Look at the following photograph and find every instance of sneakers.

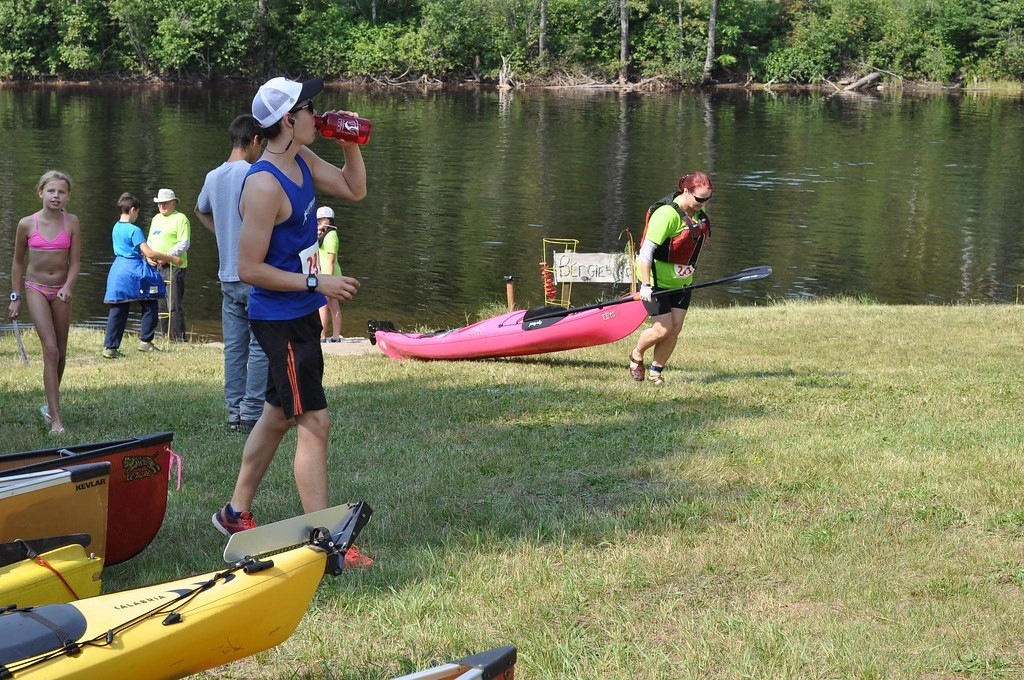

[212,502,255,540]
[343,543,374,568]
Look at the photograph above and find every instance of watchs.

[10,293,22,301]
[305,273,318,292]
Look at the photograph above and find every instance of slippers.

[48,428,65,438]
[45,413,52,426]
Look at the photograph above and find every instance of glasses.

[289,100,313,114]
[688,190,712,203]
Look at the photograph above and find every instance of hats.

[251,76,323,129]
[153,188,181,206]
[316,205,335,220]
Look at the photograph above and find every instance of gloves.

[639,287,658,303]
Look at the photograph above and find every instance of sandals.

[628,351,645,381]
[647,373,664,387]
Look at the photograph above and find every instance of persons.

[101,191,184,359]
[314,206,343,342]
[145,188,191,345]
[193,115,265,434]
[8,171,80,438]
[629,171,712,387]
[210,77,376,574]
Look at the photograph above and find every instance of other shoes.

[102,348,126,359]
[320,339,327,344]
[239,419,259,434]
[229,422,239,432]
[137,341,161,352]
[327,337,342,343]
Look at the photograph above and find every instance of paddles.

[522,266,773,332]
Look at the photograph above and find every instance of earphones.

[288,117,295,125]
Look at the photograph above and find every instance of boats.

[366,291,648,360]
[1,433,175,611]
[0,503,518,680]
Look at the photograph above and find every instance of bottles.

[315,112,373,144]
